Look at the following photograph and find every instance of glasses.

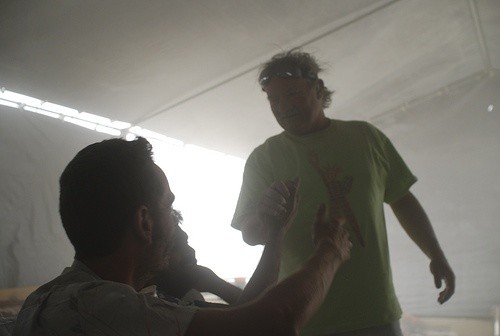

[261,70,316,91]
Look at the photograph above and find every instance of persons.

[11,136,353,336]
[231,47,456,336]
